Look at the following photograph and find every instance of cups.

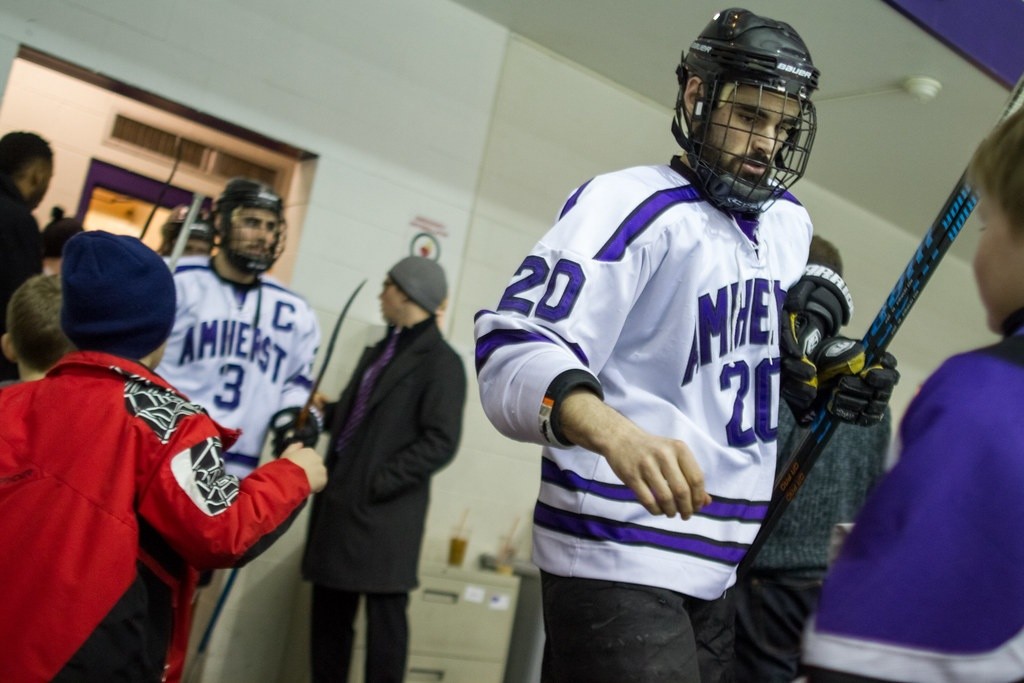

[448,528,470,566]
[497,535,521,574]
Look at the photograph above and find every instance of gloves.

[777,261,853,427]
[808,335,902,429]
[270,405,322,456]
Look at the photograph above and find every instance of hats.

[389,255,446,314]
[61,229,175,359]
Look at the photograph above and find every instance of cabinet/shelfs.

[280,560,520,683]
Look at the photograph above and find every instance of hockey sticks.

[173,278,371,683]
[694,74,1024,636]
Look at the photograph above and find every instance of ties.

[337,332,399,453]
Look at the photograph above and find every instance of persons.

[42,208,85,275]
[149,178,322,480]
[0,231,327,683]
[1,273,76,382]
[0,132,52,378]
[472,7,899,683]
[803,102,1024,683]
[727,236,891,683]
[300,256,466,683]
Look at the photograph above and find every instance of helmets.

[668,6,821,212]
[215,177,285,272]
[157,206,215,257]
